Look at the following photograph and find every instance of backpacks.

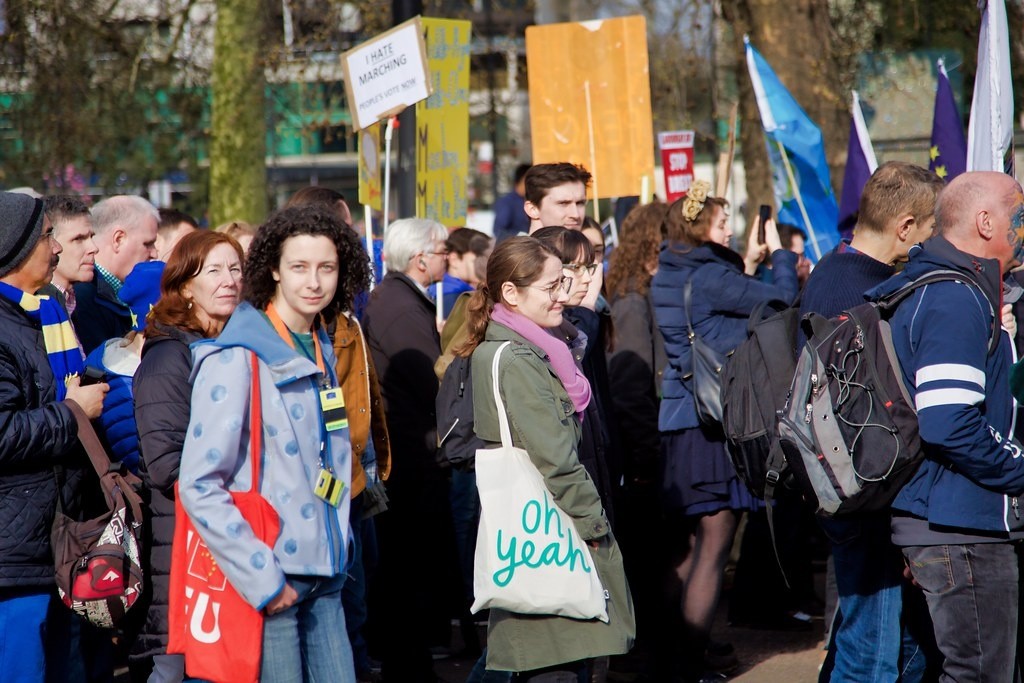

[719,248,834,500]
[763,270,996,544]
[435,354,481,465]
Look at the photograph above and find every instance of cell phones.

[758,204,771,244]
[79,365,108,387]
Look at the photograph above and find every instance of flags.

[928,57,966,180]
[745,44,879,263]
[965,0,1015,178]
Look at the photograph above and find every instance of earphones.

[420,259,427,267]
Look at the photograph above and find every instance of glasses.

[516,277,573,301]
[426,249,449,260]
[561,263,597,277]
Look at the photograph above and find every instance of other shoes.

[788,605,825,620]
[702,631,734,655]
[647,653,739,679]
[752,612,813,630]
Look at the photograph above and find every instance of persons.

[0,163,1024,683]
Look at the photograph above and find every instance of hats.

[0,192,43,277]
[6,187,44,198]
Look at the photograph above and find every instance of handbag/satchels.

[166,349,282,683]
[50,398,143,627]
[469,341,610,622]
[684,271,728,443]
